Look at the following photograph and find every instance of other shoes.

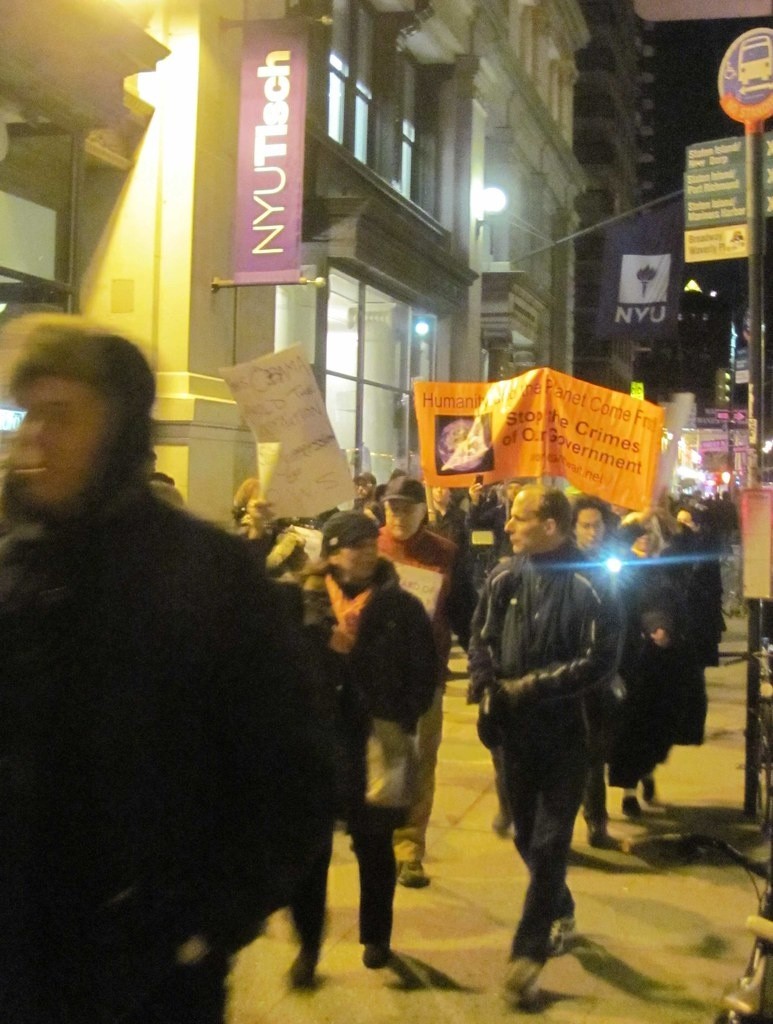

[641,772,655,802]
[362,943,389,969]
[500,955,544,1007]
[397,860,425,888]
[587,820,607,847]
[548,916,583,956]
[620,789,643,818]
[290,949,318,987]
[493,812,511,832]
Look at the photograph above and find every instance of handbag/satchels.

[366,735,425,808]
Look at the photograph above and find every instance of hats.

[320,510,382,558]
[380,476,427,505]
[352,471,377,485]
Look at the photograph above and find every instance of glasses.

[355,482,367,489]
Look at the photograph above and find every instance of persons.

[1,330,333,1023]
[233,469,742,1004]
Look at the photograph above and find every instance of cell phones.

[475,474,484,486]
